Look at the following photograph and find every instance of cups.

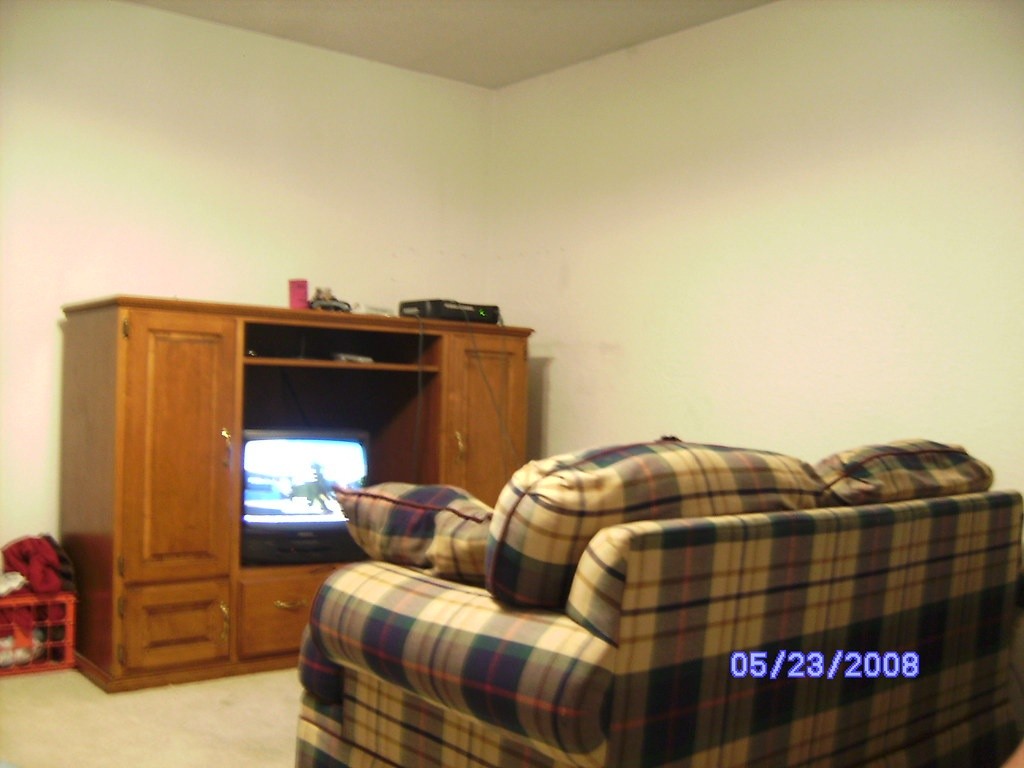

[288,278,310,311]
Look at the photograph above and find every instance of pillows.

[482,434,825,618]
[334,481,493,589]
[816,439,994,506]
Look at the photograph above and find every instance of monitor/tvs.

[240,428,373,564]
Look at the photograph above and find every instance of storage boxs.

[0,592,76,676]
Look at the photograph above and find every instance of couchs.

[295,490,1024,768]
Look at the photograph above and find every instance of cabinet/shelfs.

[59,293,535,696]
